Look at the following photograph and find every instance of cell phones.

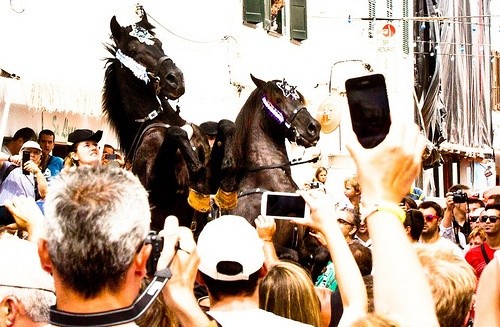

[260,191,310,221]
[345,73,391,150]
[22,151,30,176]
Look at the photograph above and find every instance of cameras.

[311,182,319,189]
[452,193,467,203]
[145,230,164,275]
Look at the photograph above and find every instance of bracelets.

[360,201,406,223]
[8,156,11,162]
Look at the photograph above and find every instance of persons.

[132,190,368,327]
[345,114,499,327]
[1,233,56,327]
[308,164,327,191]
[36,165,216,327]
[0,127,124,240]
[256,0,285,31]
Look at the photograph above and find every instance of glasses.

[337,218,354,226]
[482,215,500,223]
[136,231,157,254]
[423,214,436,222]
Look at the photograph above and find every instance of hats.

[68,128,103,144]
[20,141,42,151]
[196,215,265,281]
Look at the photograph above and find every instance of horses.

[206,72,323,271]
[97,6,240,284]
